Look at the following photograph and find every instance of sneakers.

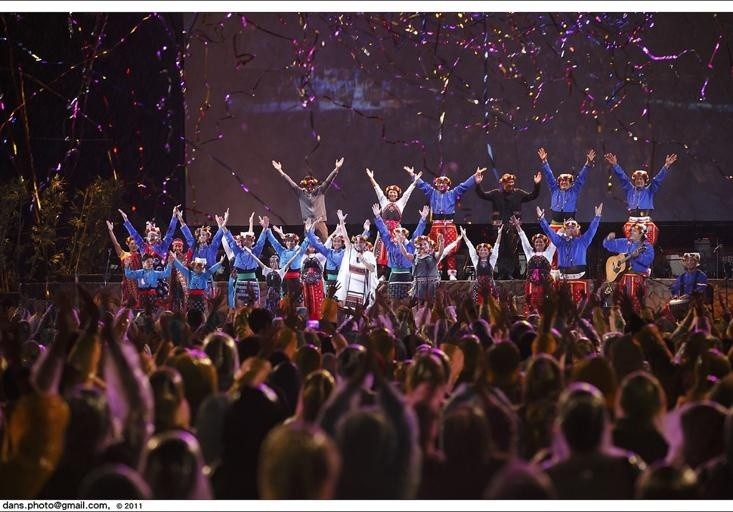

[377,274,387,283]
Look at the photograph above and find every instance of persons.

[537,147,597,269]
[364,168,422,264]
[271,157,344,242]
[403,165,487,280]
[604,153,678,243]
[473,171,541,279]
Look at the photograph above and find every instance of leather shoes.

[496,272,515,280]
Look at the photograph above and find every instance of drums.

[669,298,689,317]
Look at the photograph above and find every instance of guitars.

[605,246,646,283]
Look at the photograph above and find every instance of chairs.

[666,255,687,279]
[75,245,112,288]
[694,238,725,279]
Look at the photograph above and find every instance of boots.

[446,268,459,282]
